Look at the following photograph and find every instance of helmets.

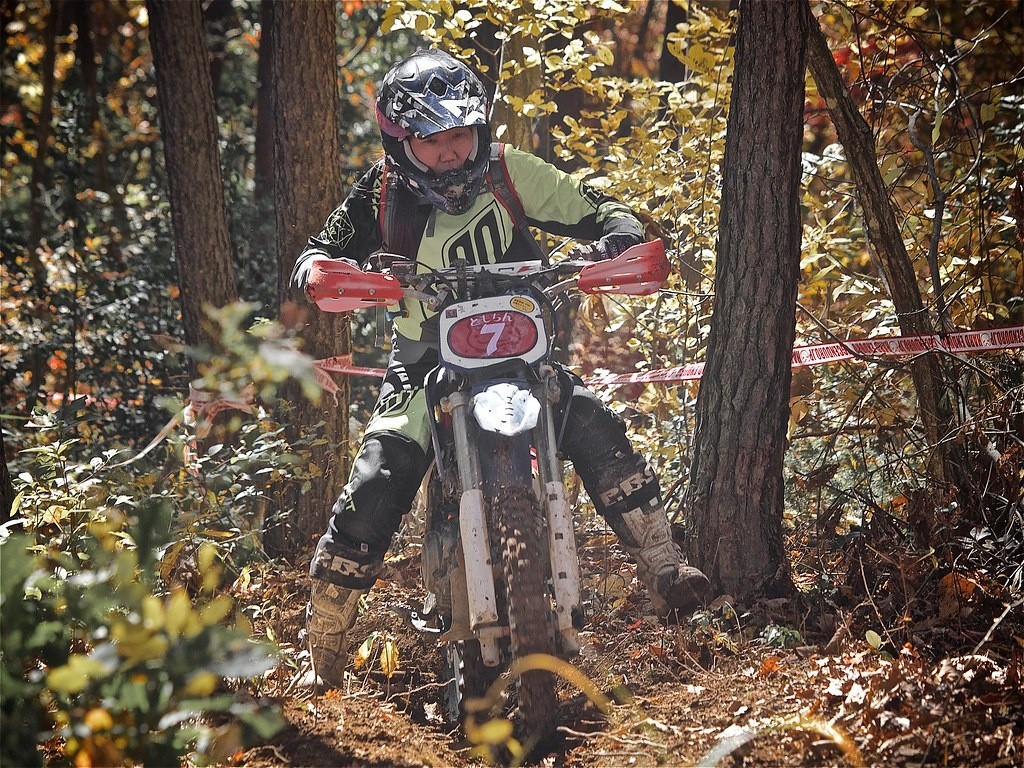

[374,48,492,216]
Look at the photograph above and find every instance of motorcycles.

[305,235,670,721]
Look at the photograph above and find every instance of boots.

[606,490,710,628]
[297,576,368,694]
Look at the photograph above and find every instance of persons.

[289,49,710,695]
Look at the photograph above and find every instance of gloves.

[336,257,359,269]
[597,233,640,261]
